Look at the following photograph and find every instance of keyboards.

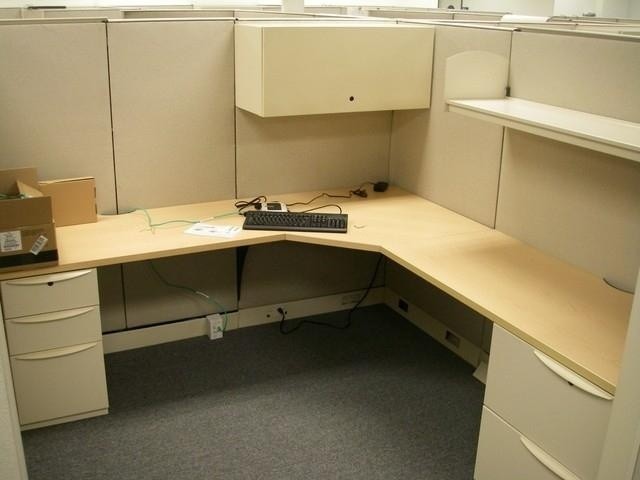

[243,211,348,232]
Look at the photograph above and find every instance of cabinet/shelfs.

[234,20,436,120]
[2,269,113,433]
[472,323,613,480]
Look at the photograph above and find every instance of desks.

[0,183,633,396]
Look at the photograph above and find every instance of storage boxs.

[1,167,59,273]
